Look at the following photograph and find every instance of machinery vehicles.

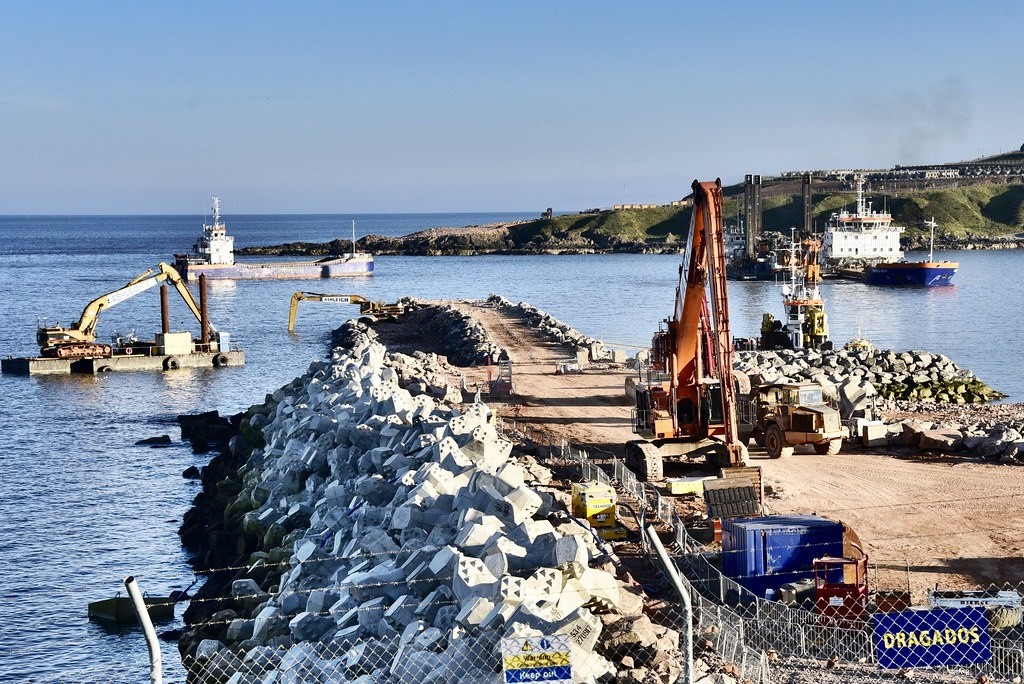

[36,261,220,358]
[288,291,390,331]
[624,175,765,513]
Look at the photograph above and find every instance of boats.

[170,197,375,281]
[817,175,960,289]
[721,172,823,286]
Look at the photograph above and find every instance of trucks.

[735,378,850,462]
